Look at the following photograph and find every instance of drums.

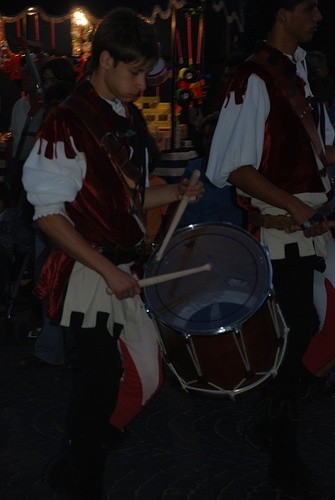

[143,219,291,398]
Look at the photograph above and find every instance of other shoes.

[45,453,106,499]
[269,455,322,500]
[245,413,267,450]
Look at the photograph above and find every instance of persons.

[21,8,205,500]
[0,32,335,401]
[204,0,335,500]
